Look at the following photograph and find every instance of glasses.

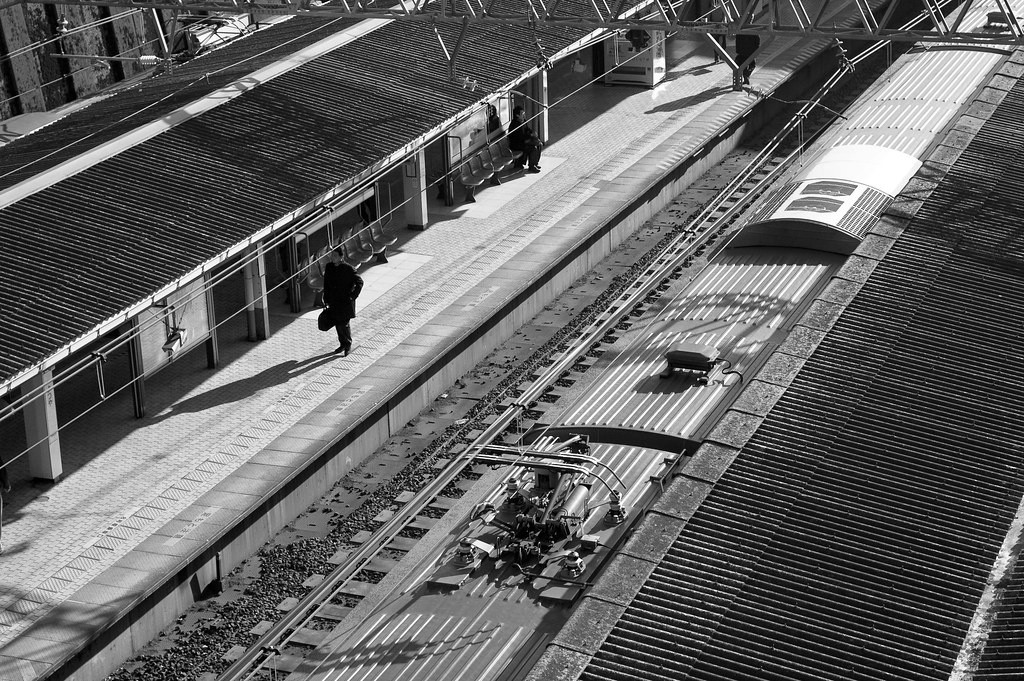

[520,112,526,115]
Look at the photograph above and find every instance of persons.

[505,104,544,175]
[317,245,362,359]
[487,105,500,136]
[713,32,729,64]
[736,30,763,87]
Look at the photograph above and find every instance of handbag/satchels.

[525,128,540,145]
[318,304,335,331]
[734,53,742,66]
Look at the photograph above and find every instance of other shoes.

[529,166,540,172]
[715,60,719,63]
[536,166,541,169]
[744,75,749,84]
[345,349,349,356]
[335,346,345,353]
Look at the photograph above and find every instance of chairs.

[370,222,398,264]
[469,157,495,179]
[498,138,523,159]
[317,253,329,276]
[458,165,484,203]
[479,150,505,185]
[344,237,374,263]
[488,144,514,165]
[306,256,326,308]
[358,229,386,254]
[330,245,361,268]
